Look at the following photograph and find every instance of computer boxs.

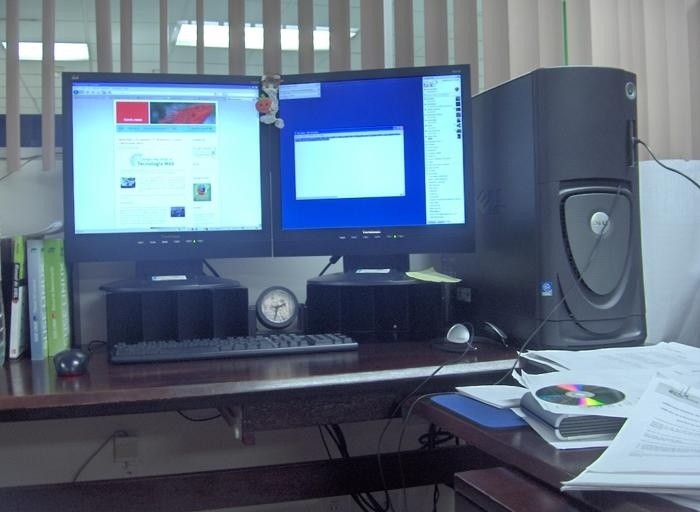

[445,66,647,349]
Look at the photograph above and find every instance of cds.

[535,383,625,409]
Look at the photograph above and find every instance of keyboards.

[108,331,361,365]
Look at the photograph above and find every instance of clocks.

[253,283,300,330]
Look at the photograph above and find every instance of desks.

[2,339,535,429]
[415,388,698,510]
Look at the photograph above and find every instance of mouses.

[53,348,90,377]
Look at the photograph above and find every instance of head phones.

[433,319,511,353]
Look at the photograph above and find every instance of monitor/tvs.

[272,64,477,286]
[60,71,273,291]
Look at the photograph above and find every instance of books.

[8,232,28,360]
[511,337,700,493]
[41,229,72,358]
[0,234,14,359]
[22,222,63,362]
[0,356,61,397]
